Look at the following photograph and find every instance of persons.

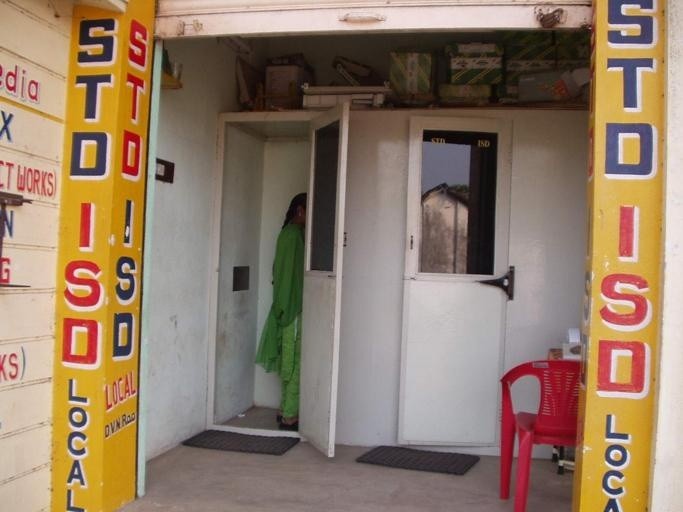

[252,192,307,431]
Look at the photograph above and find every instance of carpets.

[356,445,481,476]
[182,429,300,455]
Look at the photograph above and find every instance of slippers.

[277,411,299,431]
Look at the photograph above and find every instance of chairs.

[499,360,584,512]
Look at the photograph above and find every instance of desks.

[546,345,583,475]
